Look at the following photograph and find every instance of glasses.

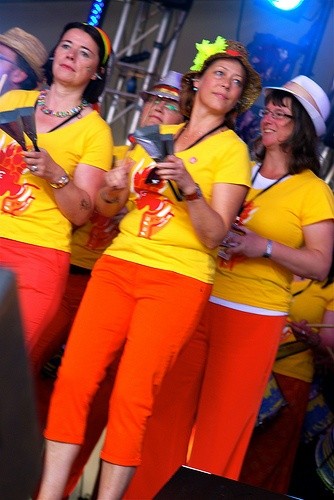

[259,108,295,120]
[148,96,179,111]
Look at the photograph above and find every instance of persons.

[119,70,333,500]
[0,23,49,93]
[236,255,333,494]
[32,29,263,500]
[0,19,116,460]
[23,68,189,499]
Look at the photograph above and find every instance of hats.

[178,35,261,119]
[0,27,49,84]
[262,74,331,138]
[139,70,188,103]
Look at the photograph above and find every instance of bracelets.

[262,237,280,264]
[48,163,71,193]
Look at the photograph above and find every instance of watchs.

[180,176,206,207]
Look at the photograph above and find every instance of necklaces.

[261,169,280,180]
[181,120,209,143]
[36,87,89,120]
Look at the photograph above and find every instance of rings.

[30,166,37,173]
[301,331,306,335]
